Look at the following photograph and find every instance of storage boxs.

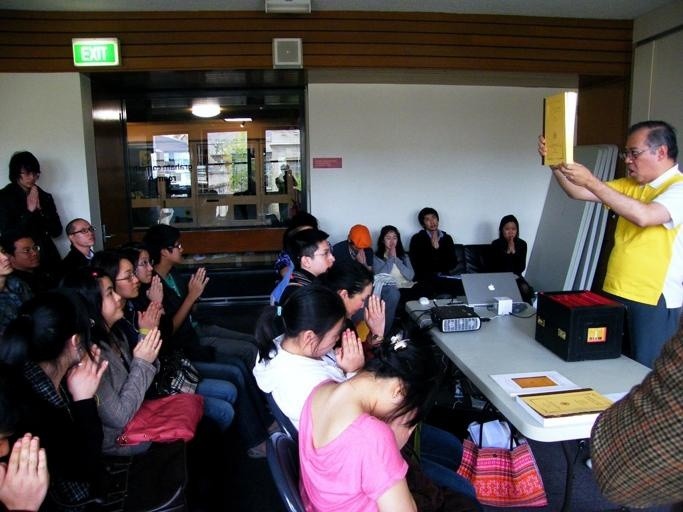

[532,287,637,362]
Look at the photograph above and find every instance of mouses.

[418,297,429,305]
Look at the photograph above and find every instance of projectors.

[430,305,481,333]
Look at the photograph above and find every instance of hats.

[348,225,373,249]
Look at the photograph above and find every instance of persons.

[408,208,464,296]
[537,120,682,368]
[487,214,533,306]
[0,150,63,269]
[1,219,474,510]
[274,163,298,219]
[589,306,681,512]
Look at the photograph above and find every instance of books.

[515,387,613,427]
[488,369,579,398]
[539,90,579,167]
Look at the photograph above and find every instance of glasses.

[16,245,41,253]
[73,226,97,234]
[163,242,185,250]
[114,270,139,282]
[135,259,155,268]
[619,146,651,159]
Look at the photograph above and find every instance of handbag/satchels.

[455,401,549,509]
[116,393,203,444]
[153,357,201,398]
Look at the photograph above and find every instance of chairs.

[264,390,299,440]
[264,427,307,512]
[464,243,493,271]
[450,242,465,276]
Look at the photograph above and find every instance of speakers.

[493,296,513,316]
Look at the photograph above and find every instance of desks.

[404,295,656,512]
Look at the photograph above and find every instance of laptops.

[457,272,524,307]
